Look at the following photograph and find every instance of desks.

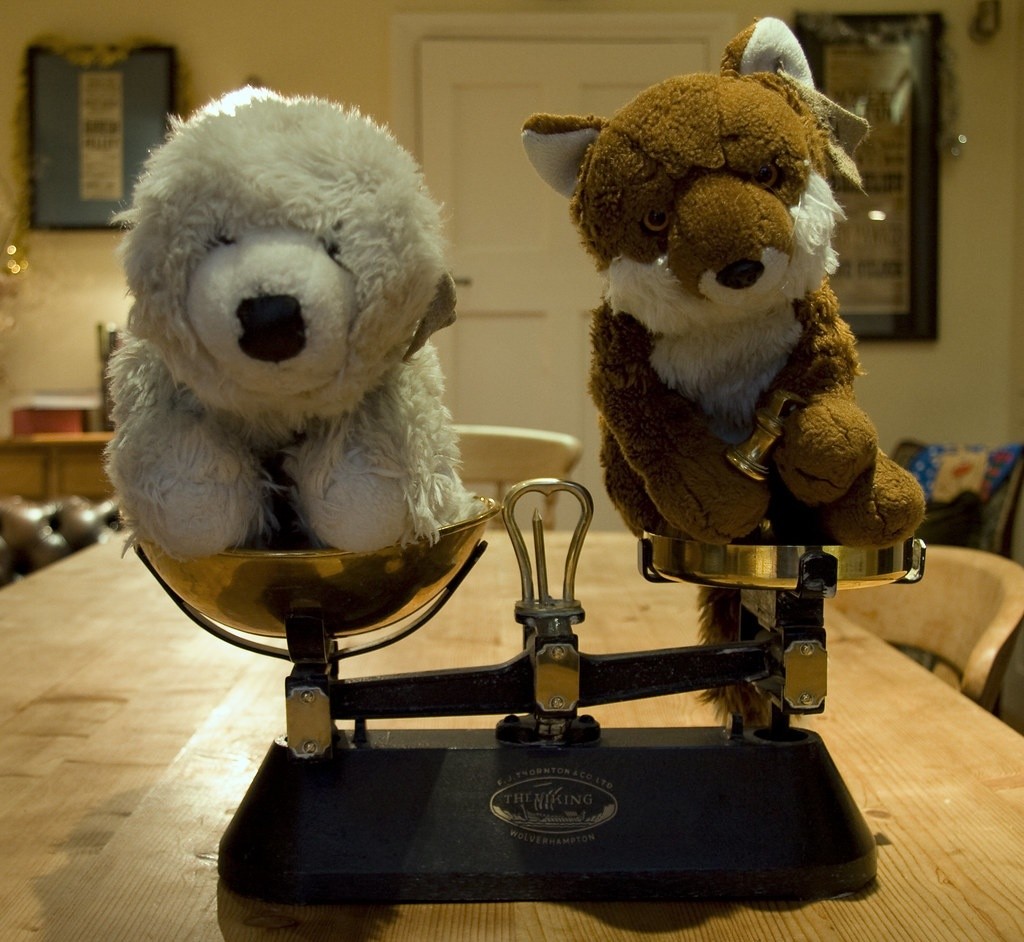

[0,531,1023,942]
[0,432,115,501]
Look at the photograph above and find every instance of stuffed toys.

[106,84,480,556]
[519,14,928,726]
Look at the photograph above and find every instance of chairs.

[449,425,582,535]
[830,545,1022,711]
[892,440,1024,556]
